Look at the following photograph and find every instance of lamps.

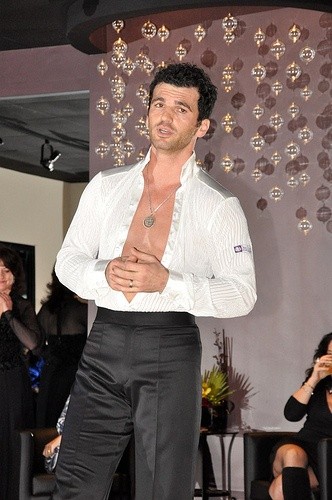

[40,138,63,171]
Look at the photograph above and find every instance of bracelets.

[302,383,315,395]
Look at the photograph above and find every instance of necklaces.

[143,161,182,229]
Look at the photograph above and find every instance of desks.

[199,429,243,500]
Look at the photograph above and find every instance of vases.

[201,401,213,429]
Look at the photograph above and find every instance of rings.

[129,277,134,287]
[48,450,50,453]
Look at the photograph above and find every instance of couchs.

[243,431,332,500]
[19,428,135,500]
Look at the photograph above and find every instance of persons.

[43,394,137,500]
[0,245,43,500]
[35,261,83,476]
[49,62,258,500]
[268,331,332,500]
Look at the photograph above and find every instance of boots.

[282,467,311,500]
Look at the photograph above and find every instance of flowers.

[200,368,235,406]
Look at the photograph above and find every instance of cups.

[325,357,332,375]
